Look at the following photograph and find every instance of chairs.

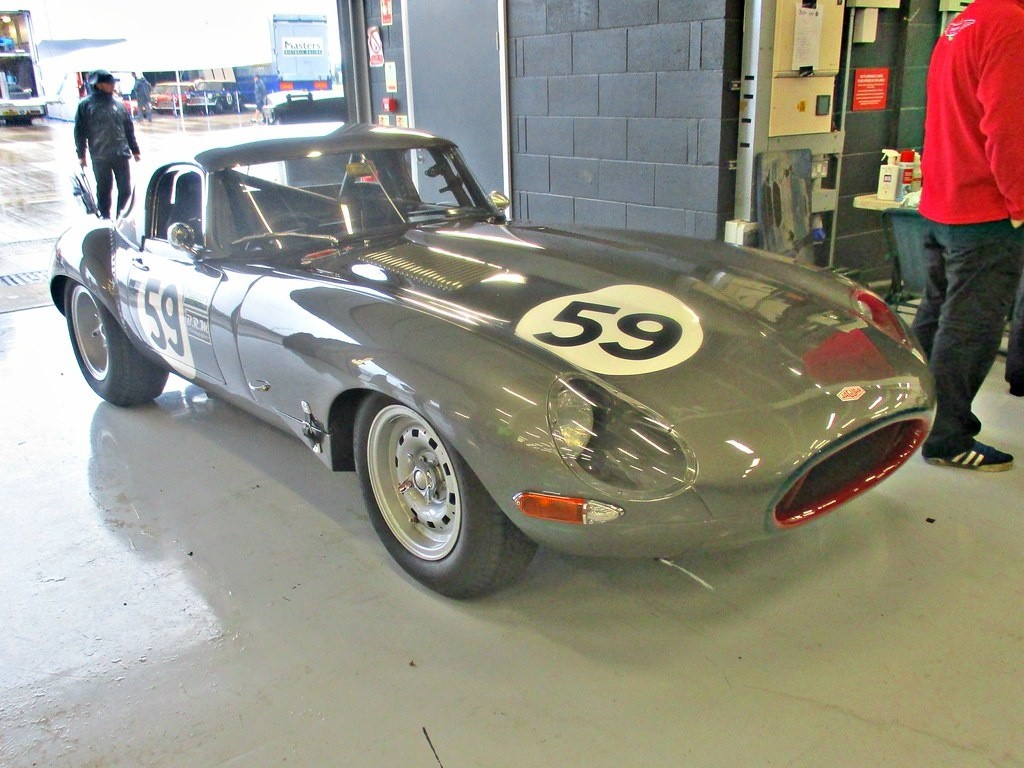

[167,170,241,257]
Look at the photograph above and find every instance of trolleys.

[880,209,937,313]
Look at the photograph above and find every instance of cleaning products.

[876,148,922,202]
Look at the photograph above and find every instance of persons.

[133,76,152,122]
[911,0,1024,471]
[73,70,140,218]
[251,74,269,122]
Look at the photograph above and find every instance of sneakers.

[923,437,1013,472]
[967,411,980,438]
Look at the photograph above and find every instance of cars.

[186,80,242,114]
[149,83,194,116]
[47,123,938,599]
[261,91,307,126]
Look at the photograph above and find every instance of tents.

[69,34,273,118]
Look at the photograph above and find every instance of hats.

[95,71,120,84]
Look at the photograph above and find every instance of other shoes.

[1010,384,1024,397]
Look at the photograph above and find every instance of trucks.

[0,10,48,126]
[43,68,154,120]
